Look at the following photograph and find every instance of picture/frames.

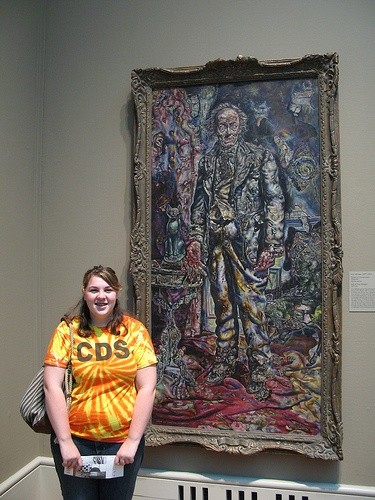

[130,52,345,462]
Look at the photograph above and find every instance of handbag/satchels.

[19,363,72,433]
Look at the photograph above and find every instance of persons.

[41,264,159,499]
